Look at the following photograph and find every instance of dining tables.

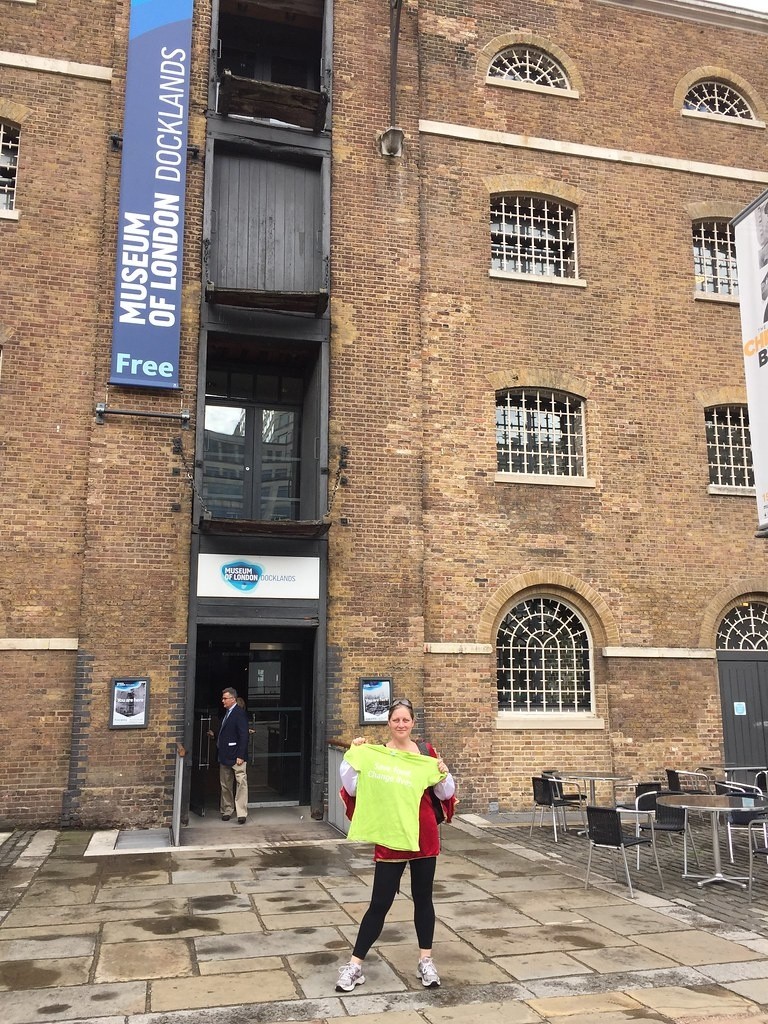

[700,765,767,826]
[654,795,768,891]
[552,771,634,837]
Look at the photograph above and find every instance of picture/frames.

[357,677,395,726]
[108,676,149,730]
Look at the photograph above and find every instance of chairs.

[529,776,589,845]
[585,805,665,901]
[616,781,664,849]
[713,779,768,850]
[665,768,721,832]
[632,790,702,879]
[539,769,588,836]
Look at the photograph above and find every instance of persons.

[207,687,250,824]
[335,699,455,993]
[126,688,134,716]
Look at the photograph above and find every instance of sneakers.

[416,956,442,987]
[335,965,366,992]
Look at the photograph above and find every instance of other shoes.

[238,817,246,823]
[222,815,230,820]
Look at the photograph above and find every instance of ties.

[222,710,230,727]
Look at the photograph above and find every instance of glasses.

[221,697,231,700]
[392,699,412,708]
